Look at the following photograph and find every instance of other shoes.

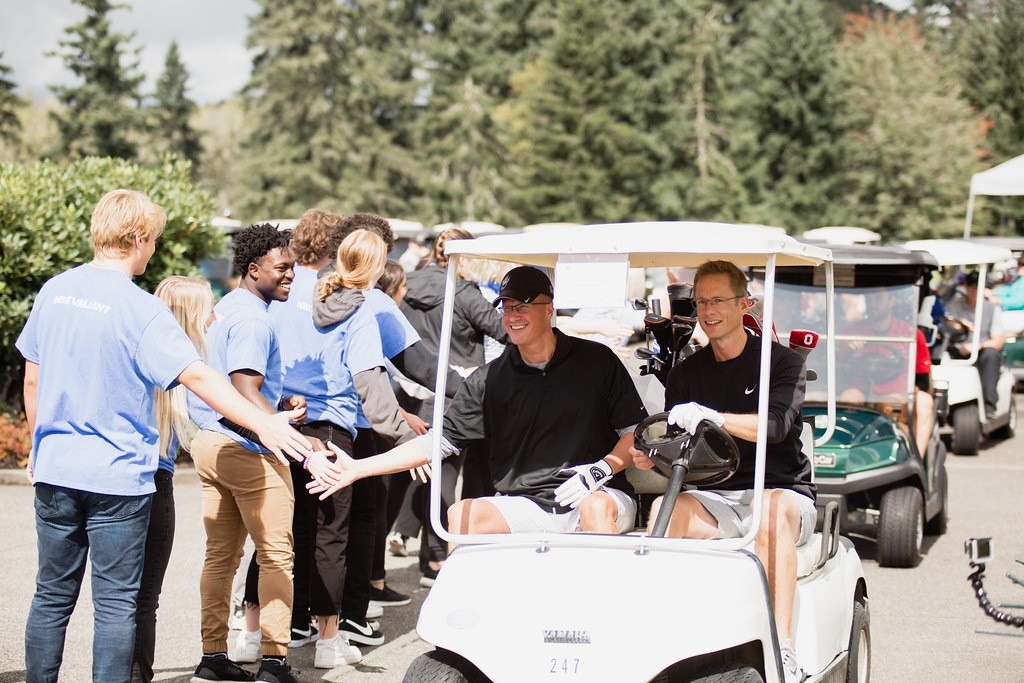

[985,402,1000,424]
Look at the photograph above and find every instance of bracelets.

[303,449,313,469]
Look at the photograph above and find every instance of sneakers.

[369,581,411,605]
[314,633,363,668]
[288,605,385,648]
[780,639,807,683]
[190,657,299,683]
[388,532,409,556]
[228,630,262,662]
[419,565,440,587]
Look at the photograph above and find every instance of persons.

[15,188,820,683]
[840,251,1024,461]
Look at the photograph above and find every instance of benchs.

[458,417,832,579]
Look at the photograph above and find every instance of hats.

[964,271,979,286]
[492,266,554,307]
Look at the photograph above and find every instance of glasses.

[498,303,550,316]
[690,295,743,310]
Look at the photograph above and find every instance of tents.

[964,154,1024,241]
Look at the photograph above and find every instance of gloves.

[554,459,614,509]
[668,402,726,435]
